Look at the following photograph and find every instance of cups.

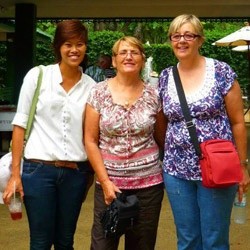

[8,192,23,220]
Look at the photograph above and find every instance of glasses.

[114,50,142,58]
[169,30,201,42]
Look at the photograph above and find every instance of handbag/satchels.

[9,137,26,158]
[198,139,244,189]
[100,192,140,238]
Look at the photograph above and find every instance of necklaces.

[110,76,141,106]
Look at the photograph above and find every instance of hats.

[0,151,24,205]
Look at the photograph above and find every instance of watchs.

[241,158,249,167]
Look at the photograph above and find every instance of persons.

[85,54,116,82]
[83,36,168,250]
[159,14,250,250]
[3,19,97,250]
[146,72,158,88]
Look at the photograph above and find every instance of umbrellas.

[211,22,250,61]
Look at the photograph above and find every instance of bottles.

[234,189,246,224]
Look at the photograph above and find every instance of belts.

[28,158,79,170]
